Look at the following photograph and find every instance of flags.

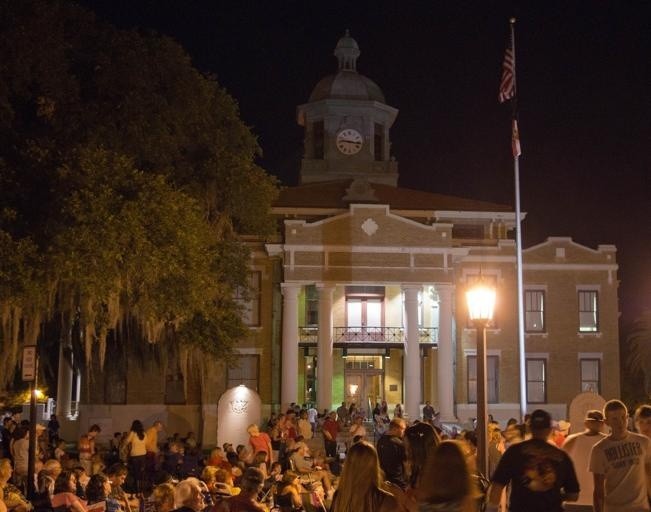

[497,26,522,158]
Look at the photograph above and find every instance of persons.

[2,386,651,512]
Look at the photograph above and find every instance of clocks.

[335,125,366,160]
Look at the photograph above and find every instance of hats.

[554,419,572,432]
[584,410,606,423]
[221,441,268,492]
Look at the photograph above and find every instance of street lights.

[460,258,501,482]
